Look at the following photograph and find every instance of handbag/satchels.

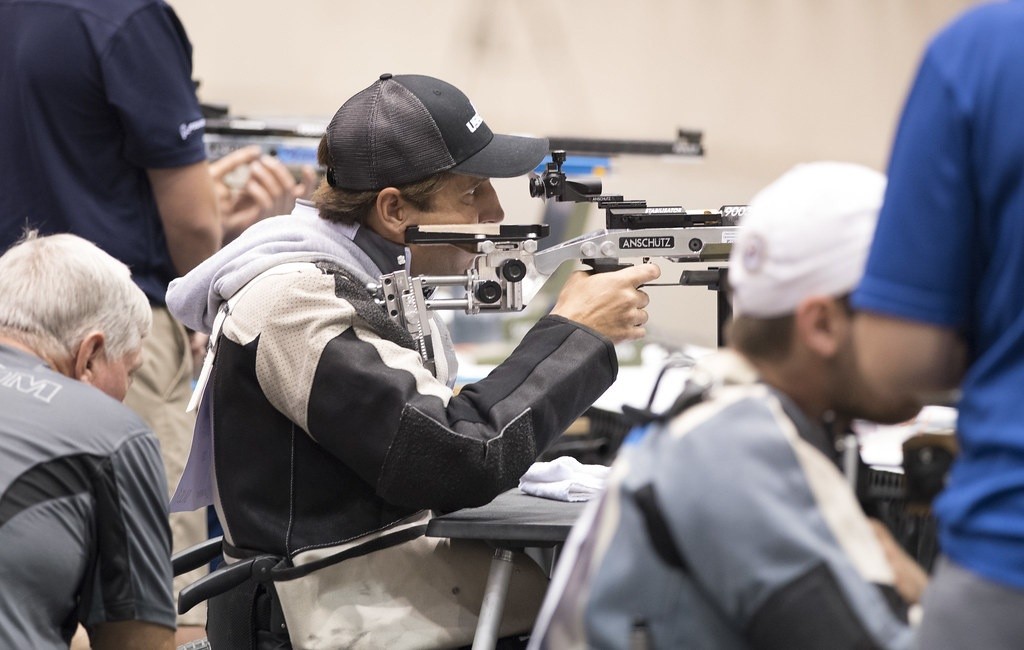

[265,511,553,648]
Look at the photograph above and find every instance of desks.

[426,458,611,650]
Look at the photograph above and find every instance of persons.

[0,0,659,650]
[849,0,1024,650]
[528,160,930,650]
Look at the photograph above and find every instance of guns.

[368,147,753,344]
[835,432,954,577]
[196,116,707,196]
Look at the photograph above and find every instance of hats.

[726,159,890,318]
[321,74,551,197]
